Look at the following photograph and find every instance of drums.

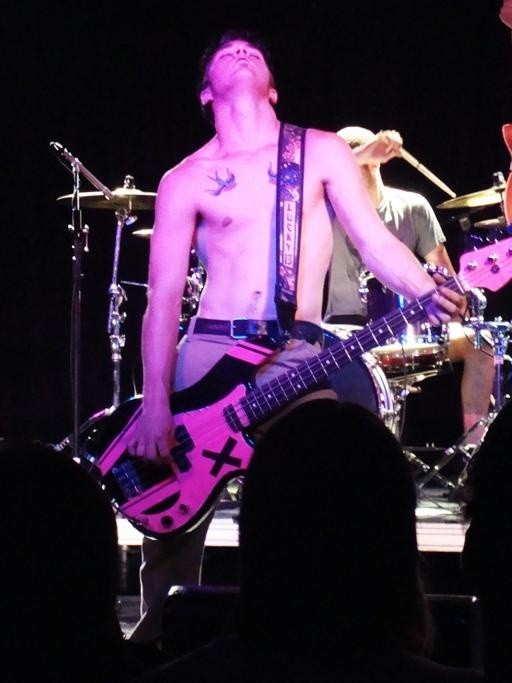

[376,347,449,386]
[225,321,402,503]
[358,262,450,355]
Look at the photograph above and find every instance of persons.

[119,27,470,621]
[2,393,512,683]
[319,123,496,461]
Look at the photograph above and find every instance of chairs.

[161,582,486,679]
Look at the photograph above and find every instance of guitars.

[84,236,512,541]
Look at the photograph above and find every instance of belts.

[193,317,321,345]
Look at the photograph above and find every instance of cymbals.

[476,216,507,227]
[133,227,154,241]
[436,183,508,210]
[56,186,157,211]
